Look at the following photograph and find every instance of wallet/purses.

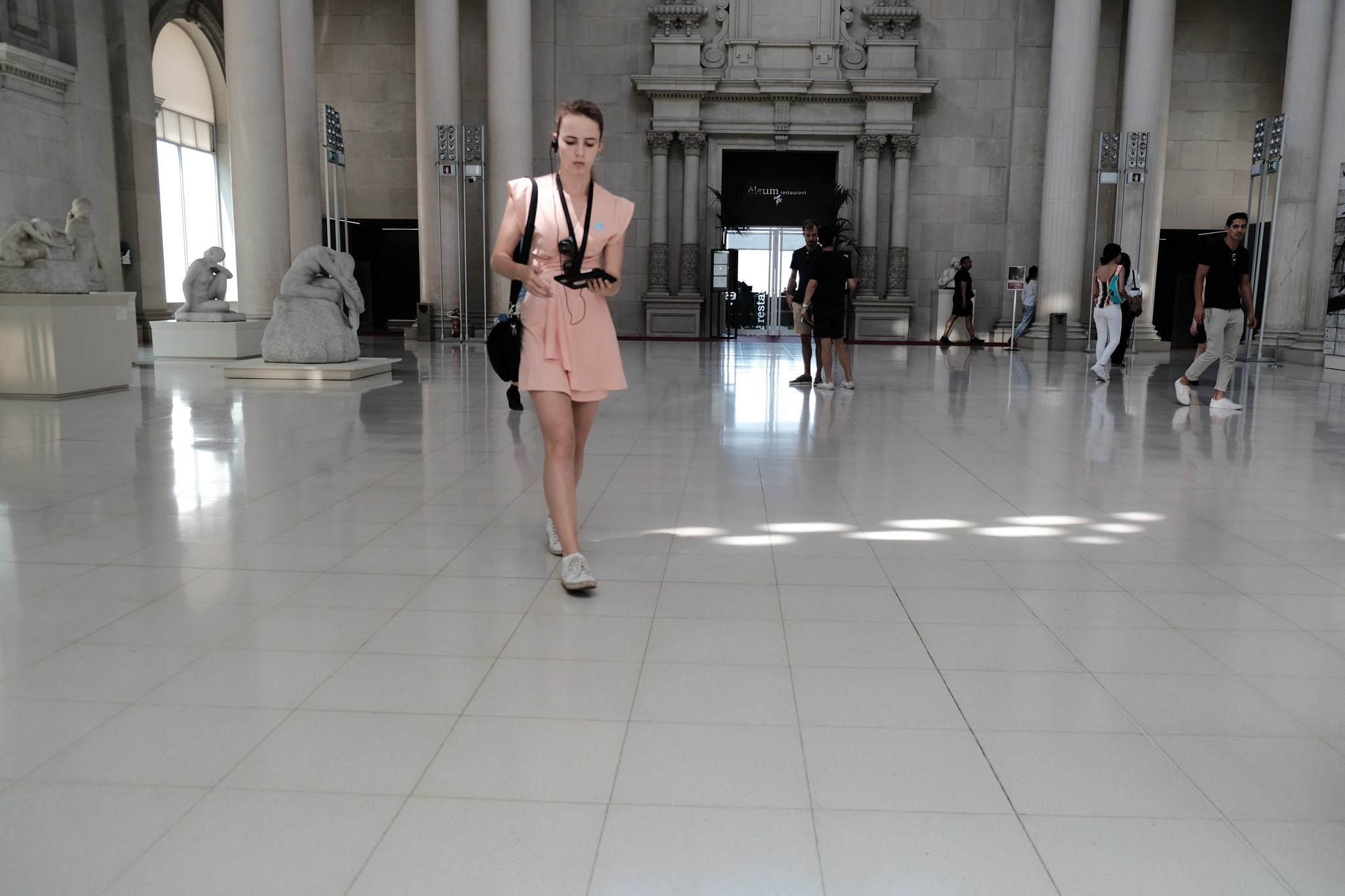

[801,317,814,329]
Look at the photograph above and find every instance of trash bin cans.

[1048,312,1067,352]
[417,302,435,341]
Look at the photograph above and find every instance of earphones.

[551,138,558,149]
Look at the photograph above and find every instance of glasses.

[966,260,973,264]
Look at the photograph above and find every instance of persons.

[506,230,526,413]
[800,225,858,389]
[786,219,825,388]
[1172,214,1256,409]
[281,245,364,341]
[1008,265,1038,344]
[939,256,985,347]
[0,217,71,267]
[491,102,635,597]
[938,257,960,287]
[176,247,233,313]
[64,197,106,283]
[1091,243,1142,381]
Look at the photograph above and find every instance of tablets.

[554,267,617,289]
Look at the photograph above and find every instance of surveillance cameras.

[469,176,476,183]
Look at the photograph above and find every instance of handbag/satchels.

[1131,287,1143,316]
[1108,264,1125,304]
[487,315,522,382]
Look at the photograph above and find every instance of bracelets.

[802,303,807,307]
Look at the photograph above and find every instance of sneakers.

[1171,406,1189,431]
[1090,364,1110,382]
[561,552,597,589]
[813,375,823,385]
[1209,397,1242,410]
[1089,380,1110,397]
[1209,408,1243,419]
[1174,376,1191,405]
[789,375,812,384]
[841,377,854,389]
[817,380,834,390]
[545,518,563,555]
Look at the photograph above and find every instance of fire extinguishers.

[446,309,460,337]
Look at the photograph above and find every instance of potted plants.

[810,181,865,295]
[706,185,751,291]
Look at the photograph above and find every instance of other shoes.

[939,336,953,344]
[1189,380,1199,386]
[1008,338,1017,346]
[1112,362,1126,367]
[970,337,985,344]
[506,384,524,410]
